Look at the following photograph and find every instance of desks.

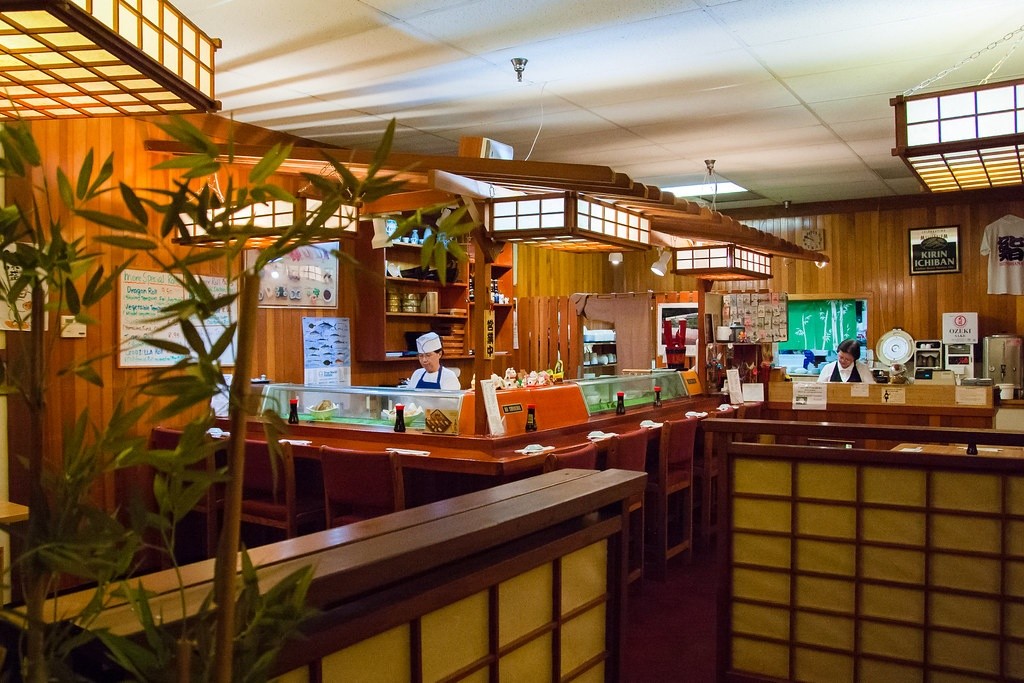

[890,444,1024,460]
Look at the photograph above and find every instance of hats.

[416,332,442,355]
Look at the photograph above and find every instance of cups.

[920,344,931,349]
[583,352,617,365]
[410,238,416,244]
[418,239,425,245]
[876,376,888,383]
[402,237,410,243]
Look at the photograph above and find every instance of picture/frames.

[242,242,339,310]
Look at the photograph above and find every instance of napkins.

[718,404,738,411]
[639,420,664,429]
[206,428,232,438]
[514,444,555,456]
[685,410,708,419]
[587,430,619,441]
[384,447,431,458]
[277,439,312,446]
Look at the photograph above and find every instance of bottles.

[288,399,299,424]
[423,225,432,245]
[490,279,499,303]
[653,387,662,407]
[616,392,626,413]
[412,230,420,244]
[260,375,266,380]
[469,279,475,301]
[918,355,939,366]
[707,385,711,397]
[394,404,406,432]
[525,404,537,432]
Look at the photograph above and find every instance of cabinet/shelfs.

[915,340,974,380]
[354,213,513,362]
[711,342,772,390]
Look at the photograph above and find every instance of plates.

[251,380,271,384]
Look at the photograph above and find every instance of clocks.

[801,229,825,251]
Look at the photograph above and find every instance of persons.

[816,338,876,383]
[406,332,461,411]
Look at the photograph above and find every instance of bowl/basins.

[207,427,223,433]
[524,444,544,450]
[640,420,655,425]
[306,402,338,419]
[719,403,730,408]
[588,430,605,436]
[382,402,424,425]
[685,411,697,416]
[584,373,596,379]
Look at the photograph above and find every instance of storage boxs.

[942,311,978,344]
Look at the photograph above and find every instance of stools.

[542,402,765,590]
[152,426,404,558]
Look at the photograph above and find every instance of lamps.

[483,182,654,255]
[670,160,775,282]
[651,246,672,277]
[172,162,363,250]
[890,24,1024,195]
[608,252,623,265]
[0,1,223,120]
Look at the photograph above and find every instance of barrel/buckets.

[994,383,1015,399]
[729,322,745,342]
